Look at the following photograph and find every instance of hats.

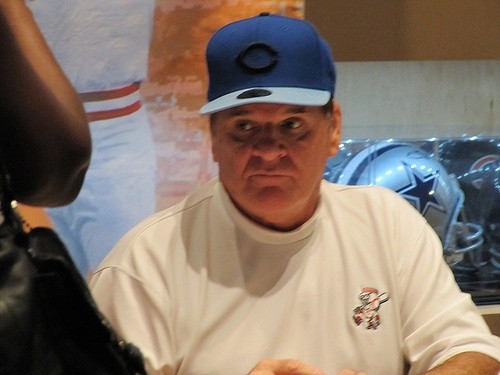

[197,12,336,115]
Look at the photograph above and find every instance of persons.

[23,0,162,291]
[84,9,499,375]
[0,0,94,273]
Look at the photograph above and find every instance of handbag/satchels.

[0,197,148,375]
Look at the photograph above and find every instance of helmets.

[321,140,484,274]
